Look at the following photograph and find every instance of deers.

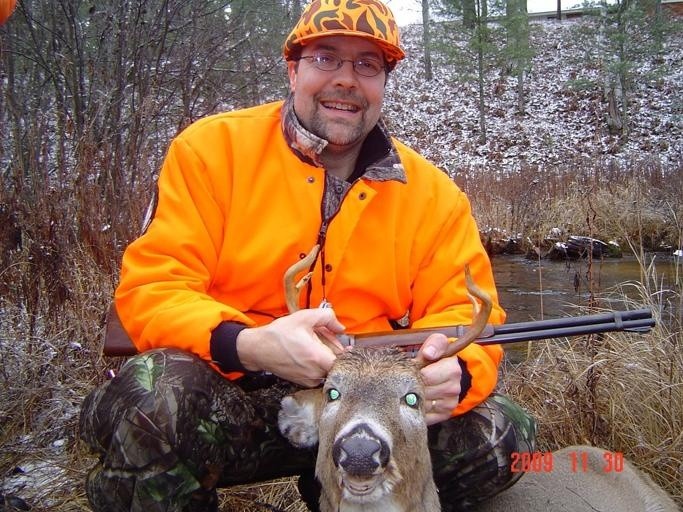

[277,243,683,512]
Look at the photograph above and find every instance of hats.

[283,0,406,62]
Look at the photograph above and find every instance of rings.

[429,399,437,413]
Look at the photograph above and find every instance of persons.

[73,0,538,510]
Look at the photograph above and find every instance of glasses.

[297,52,386,77]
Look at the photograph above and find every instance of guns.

[103,301,655,381]
